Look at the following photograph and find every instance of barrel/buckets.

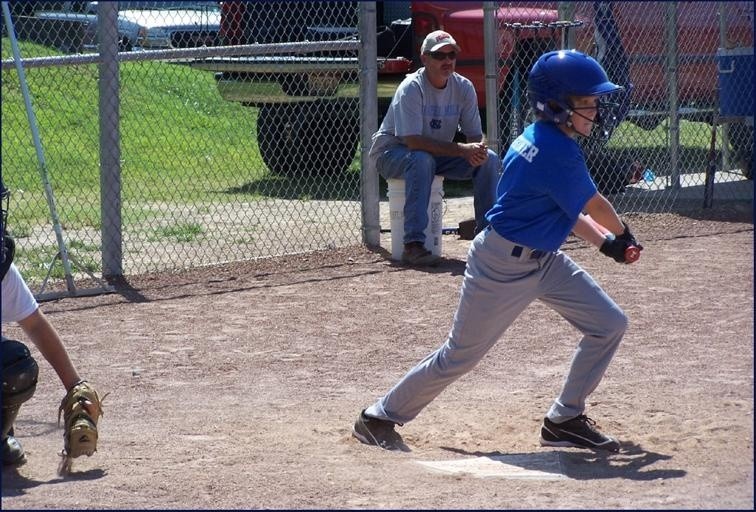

[385,176,448,259]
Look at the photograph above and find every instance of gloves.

[615,221,644,252]
[599,237,636,264]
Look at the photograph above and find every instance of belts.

[485,225,553,260]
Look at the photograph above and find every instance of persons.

[367,29,502,266]
[352,50,642,452]
[0,178,100,473]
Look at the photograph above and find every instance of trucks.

[208,0,756,186]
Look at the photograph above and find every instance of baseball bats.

[585,213,640,263]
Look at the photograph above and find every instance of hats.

[420,30,461,56]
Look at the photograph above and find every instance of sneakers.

[352,408,413,452]
[539,413,621,453]
[401,242,442,266]
[0,435,24,465]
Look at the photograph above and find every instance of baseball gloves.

[58,382,104,458]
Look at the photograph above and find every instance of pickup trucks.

[36,1,217,53]
[0,3,89,59]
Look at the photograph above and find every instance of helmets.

[525,48,625,141]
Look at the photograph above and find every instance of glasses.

[423,50,458,61]
